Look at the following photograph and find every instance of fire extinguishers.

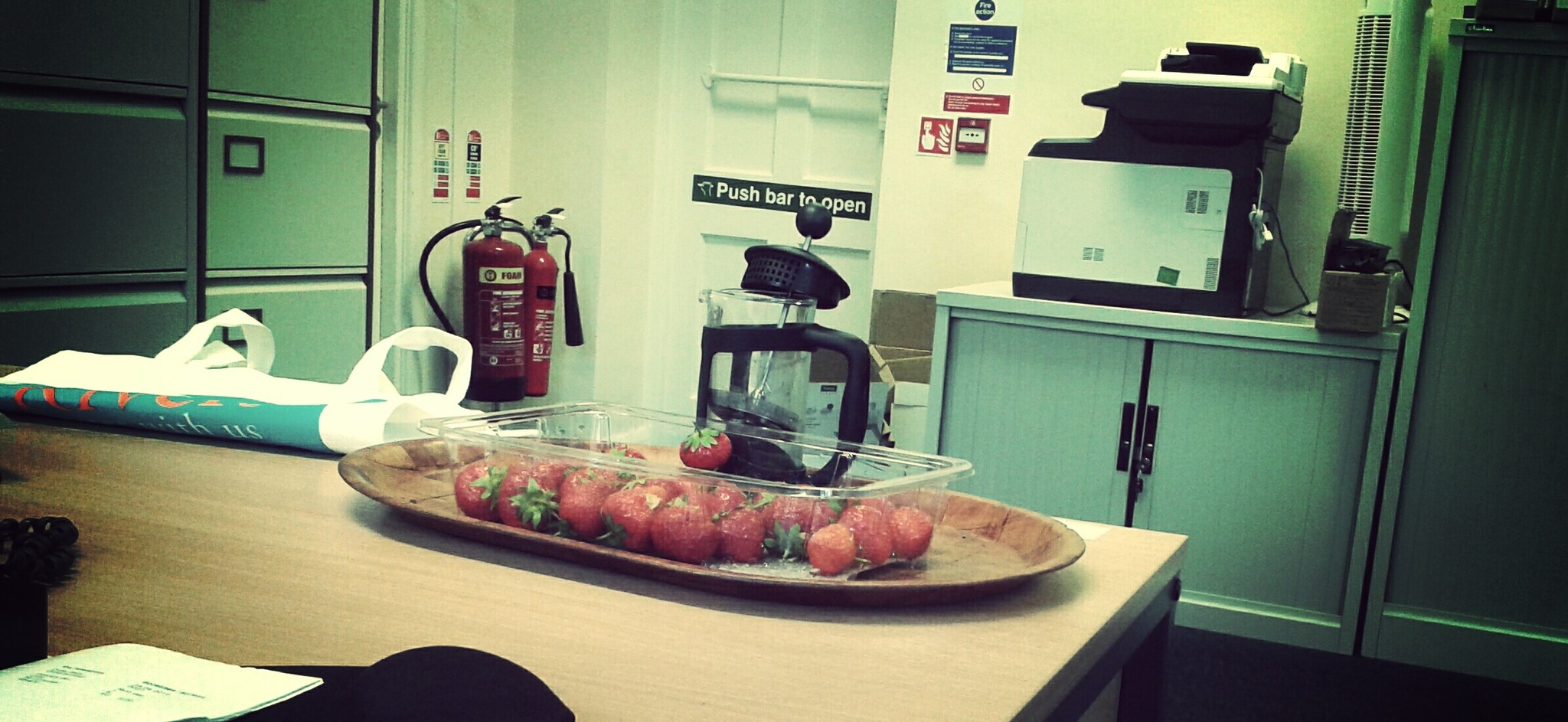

[419,196,528,403]
[524,211,584,396]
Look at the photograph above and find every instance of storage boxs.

[809,290,938,479]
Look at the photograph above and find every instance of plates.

[338,437,1087,604]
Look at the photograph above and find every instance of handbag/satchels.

[0,309,488,453]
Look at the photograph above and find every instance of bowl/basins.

[416,400,975,580]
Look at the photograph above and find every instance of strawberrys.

[679,423,731,470]
[454,444,935,574]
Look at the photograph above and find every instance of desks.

[0,361,1191,722]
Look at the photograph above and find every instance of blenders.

[695,202,870,496]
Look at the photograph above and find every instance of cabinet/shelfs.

[921,302,1398,656]
